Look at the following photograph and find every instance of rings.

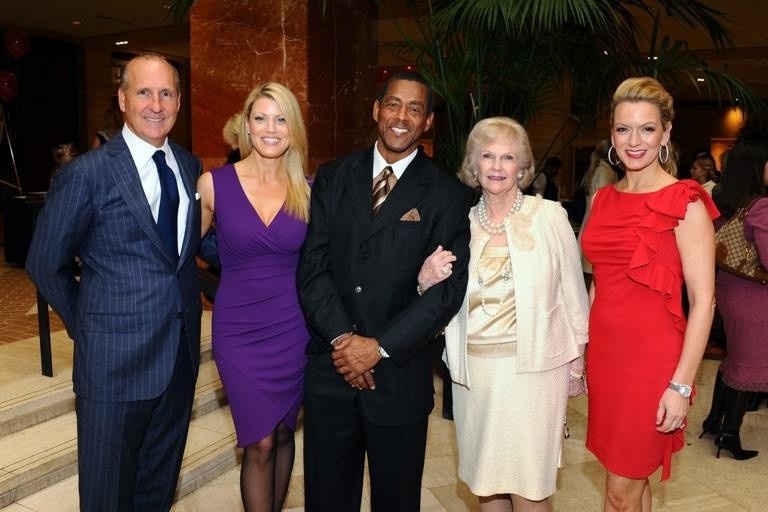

[442,265,451,276]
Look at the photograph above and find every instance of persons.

[91,108,122,152]
[293,66,470,511]
[575,77,724,512]
[220,110,250,168]
[699,120,768,462]
[529,137,737,365]
[416,114,593,512]
[196,81,314,511]
[26,52,222,512]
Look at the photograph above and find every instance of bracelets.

[416,285,424,297]
[569,371,585,380]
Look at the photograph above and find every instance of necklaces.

[475,254,513,317]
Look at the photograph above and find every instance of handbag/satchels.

[714,193,768,286]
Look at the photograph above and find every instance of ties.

[372,166,391,214]
[152,151,179,264]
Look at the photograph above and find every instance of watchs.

[378,344,390,360]
[667,381,693,398]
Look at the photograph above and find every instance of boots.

[699,371,730,439]
[715,386,759,461]
